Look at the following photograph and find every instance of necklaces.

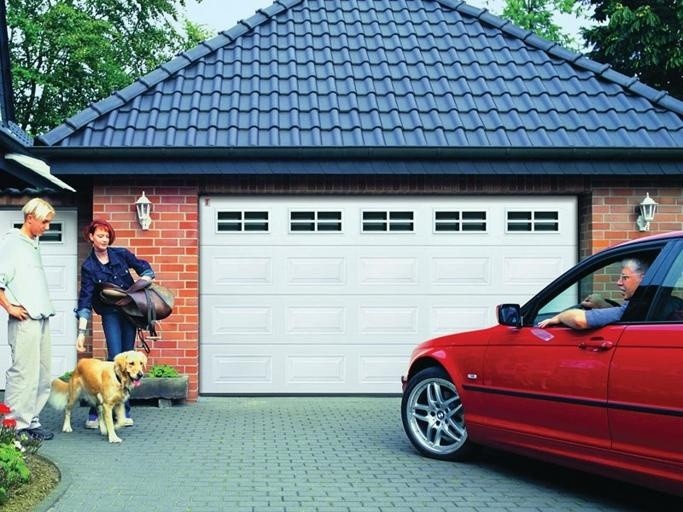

[95,250,108,256]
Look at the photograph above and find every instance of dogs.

[47,350,147,443]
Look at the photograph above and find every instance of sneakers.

[15,426,54,446]
[115,415,134,426]
[85,417,98,429]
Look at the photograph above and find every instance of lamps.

[134,190,154,231]
[636,192,660,233]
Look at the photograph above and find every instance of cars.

[395,230,683,501]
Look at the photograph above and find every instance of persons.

[0,196,55,445]
[73,220,155,429]
[536,255,652,332]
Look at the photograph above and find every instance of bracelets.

[77,328,86,337]
